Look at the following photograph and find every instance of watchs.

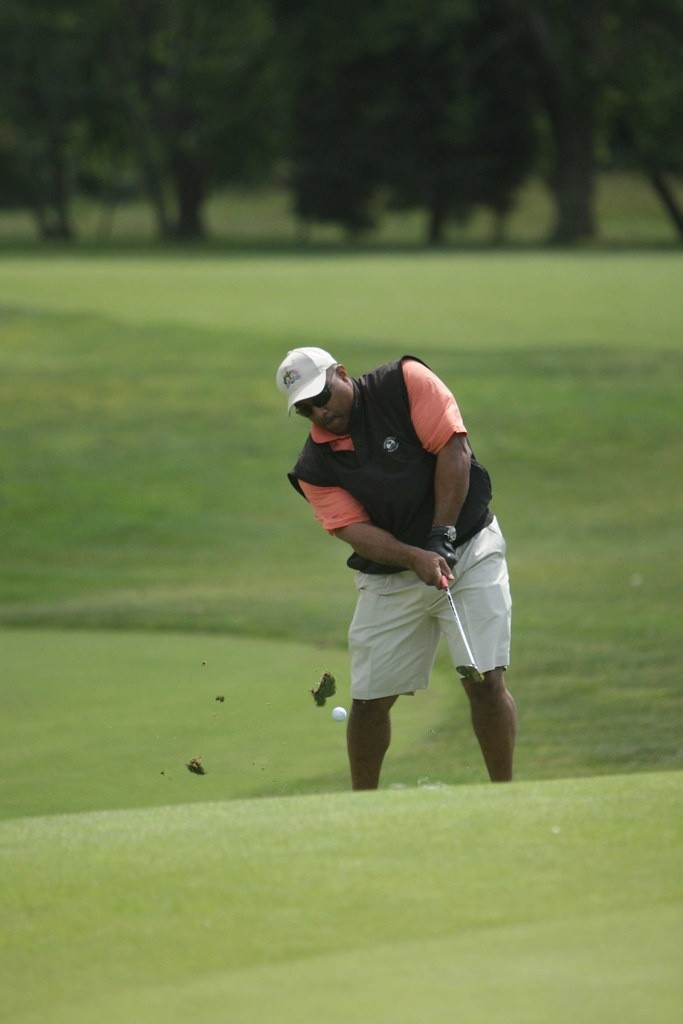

[428,525,457,542]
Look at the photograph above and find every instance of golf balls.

[332,706,348,722]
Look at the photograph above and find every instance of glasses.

[295,368,337,418]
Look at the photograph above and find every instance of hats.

[275,346,339,417]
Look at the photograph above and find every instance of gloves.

[427,530,457,571]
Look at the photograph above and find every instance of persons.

[275,347,516,792]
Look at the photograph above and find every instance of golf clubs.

[442,575,485,685]
